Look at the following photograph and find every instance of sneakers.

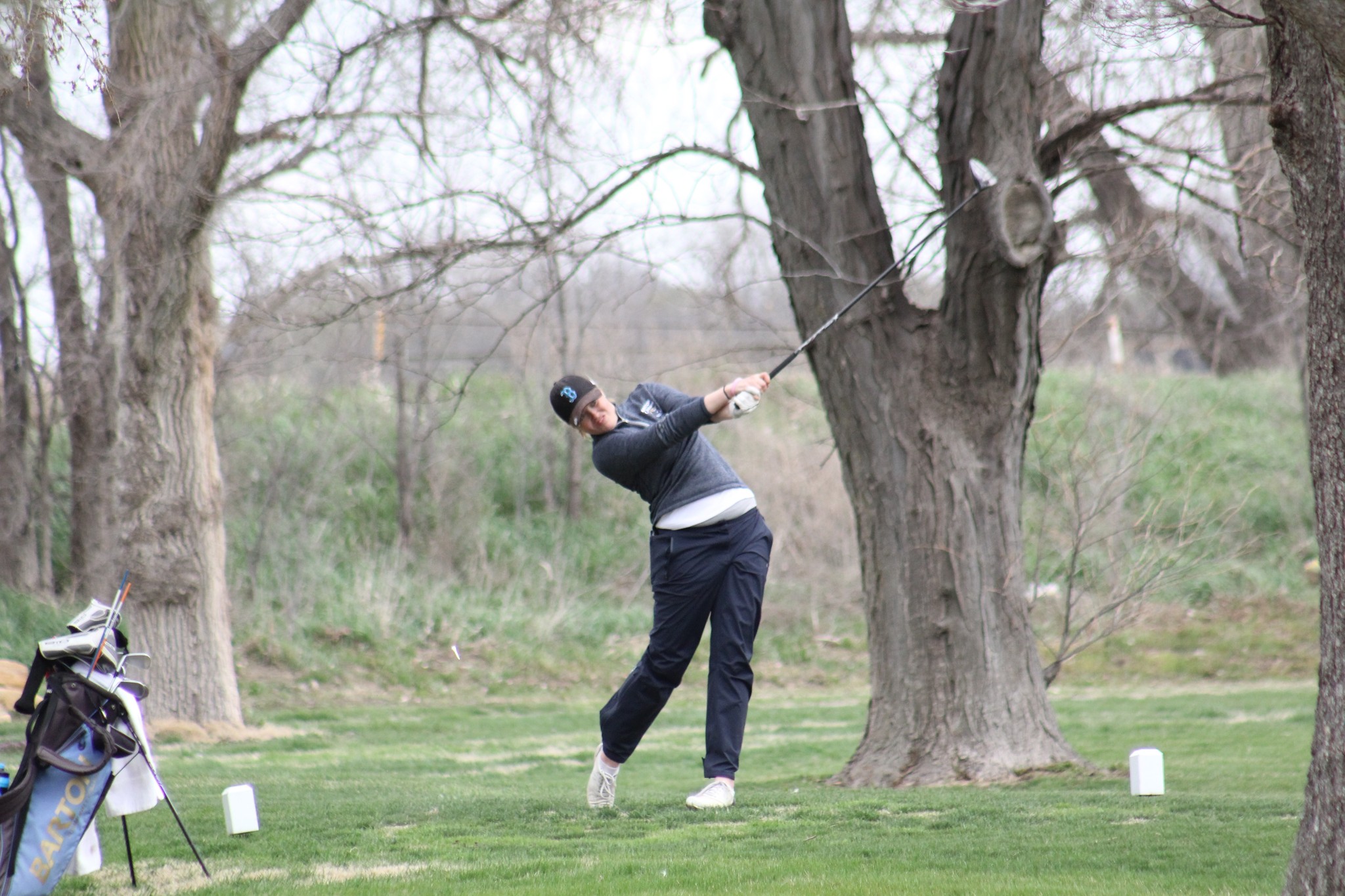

[686,781,736,811]
[587,742,620,811]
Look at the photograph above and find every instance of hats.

[550,375,602,427]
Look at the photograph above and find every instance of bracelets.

[723,378,739,399]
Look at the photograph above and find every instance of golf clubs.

[734,158,998,410]
[108,653,151,691]
[111,680,145,699]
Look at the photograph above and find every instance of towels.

[63,660,166,876]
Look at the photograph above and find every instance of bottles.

[0,763,10,796]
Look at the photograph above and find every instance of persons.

[550,373,774,810]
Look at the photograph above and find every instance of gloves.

[729,386,761,419]
